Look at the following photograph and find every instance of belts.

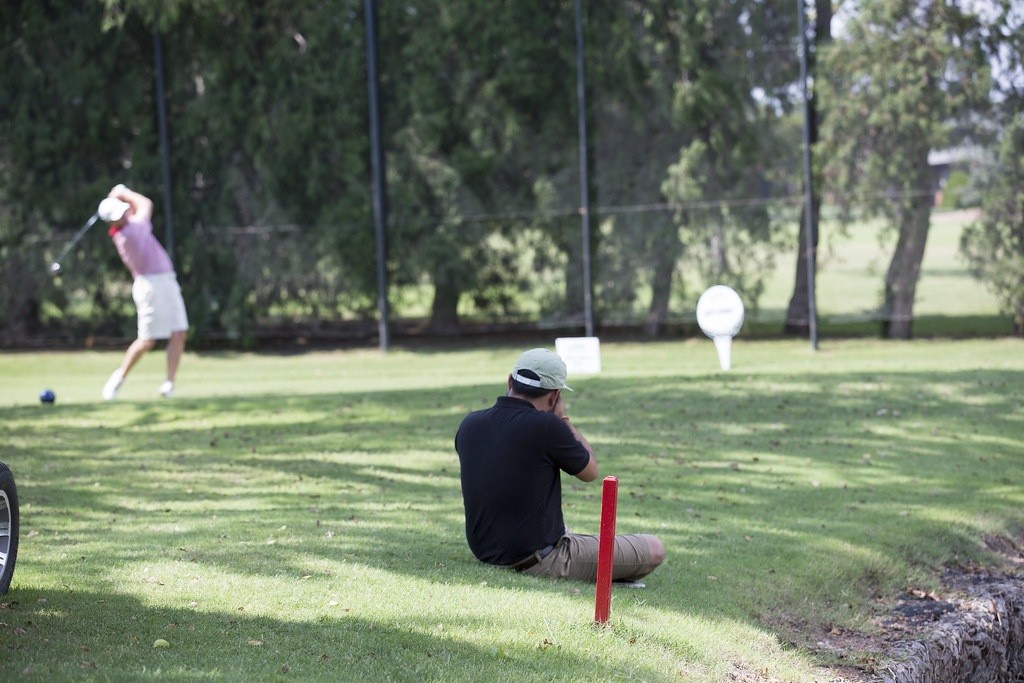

[509,542,556,572]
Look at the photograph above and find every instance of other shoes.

[160,380,177,397]
[103,369,125,399]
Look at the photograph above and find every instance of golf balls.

[696,284,744,339]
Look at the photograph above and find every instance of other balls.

[40,389,55,404]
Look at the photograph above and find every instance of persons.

[455,348,664,588]
[98,183,188,398]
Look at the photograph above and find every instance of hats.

[512,348,574,392]
[97,196,131,223]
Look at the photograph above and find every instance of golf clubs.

[46,211,99,278]
